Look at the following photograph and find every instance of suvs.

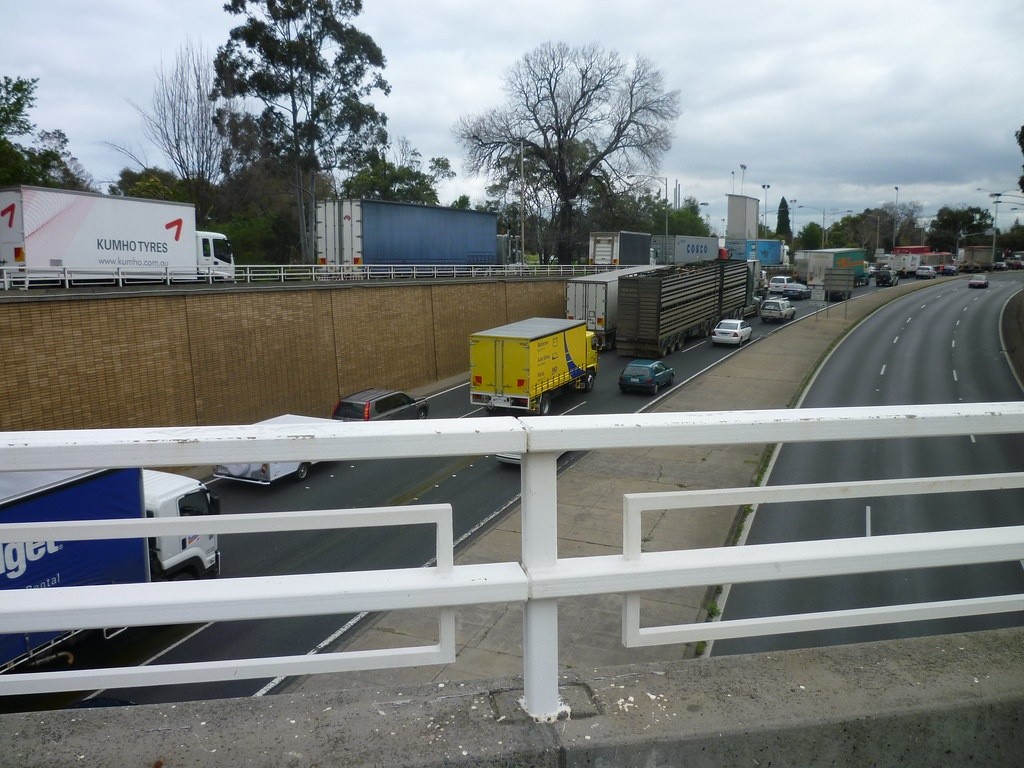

[333,387,430,421]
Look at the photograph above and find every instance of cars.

[618,360,675,396]
[759,284,811,322]
[712,319,753,348]
[866,259,1024,289]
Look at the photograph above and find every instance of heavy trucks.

[563,263,667,349]
[807,250,871,288]
[615,258,763,358]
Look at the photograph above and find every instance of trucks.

[0,467,224,674]
[313,199,513,281]
[724,237,791,268]
[651,233,726,266]
[470,317,602,415]
[1,184,235,287]
[588,230,659,271]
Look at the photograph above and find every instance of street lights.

[975,185,1020,261]
[788,186,899,252]
[627,174,669,265]
[520,198,578,277]
[760,184,771,237]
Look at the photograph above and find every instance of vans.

[768,276,797,294]
